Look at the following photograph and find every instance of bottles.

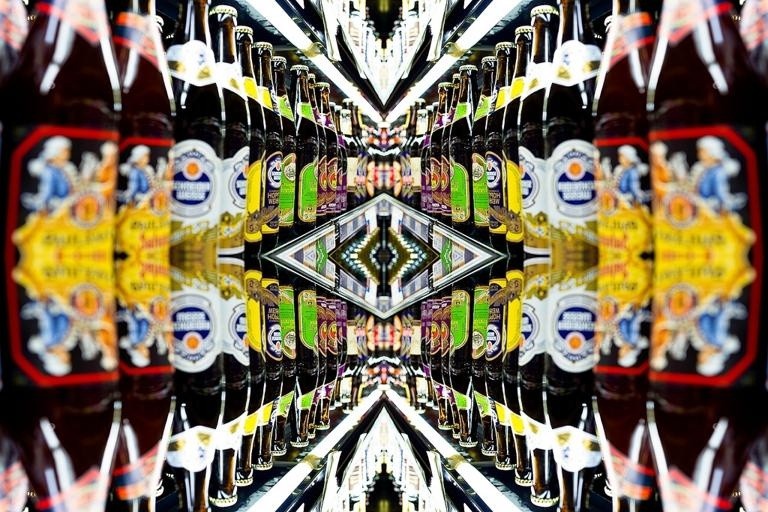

[1,0,766,511]
